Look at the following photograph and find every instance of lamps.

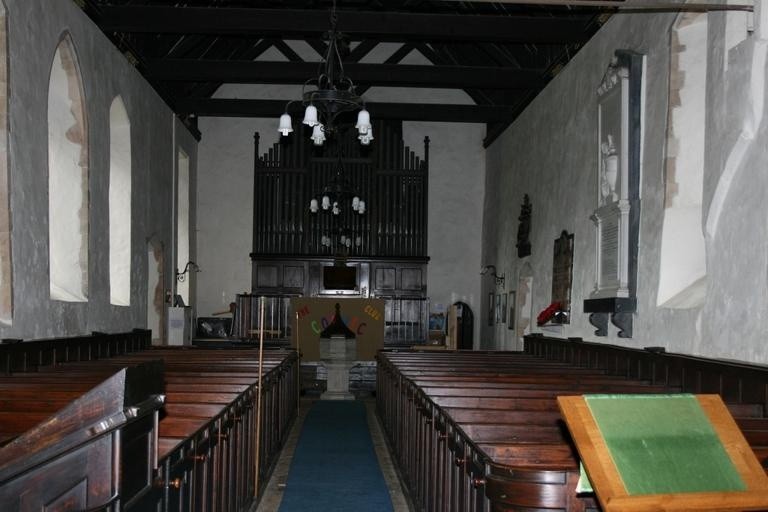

[276,1,374,146]
[175,260,202,286]
[479,264,505,290]
[321,215,362,248]
[310,127,369,216]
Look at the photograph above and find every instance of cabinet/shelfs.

[376,268,423,291]
[257,263,305,288]
